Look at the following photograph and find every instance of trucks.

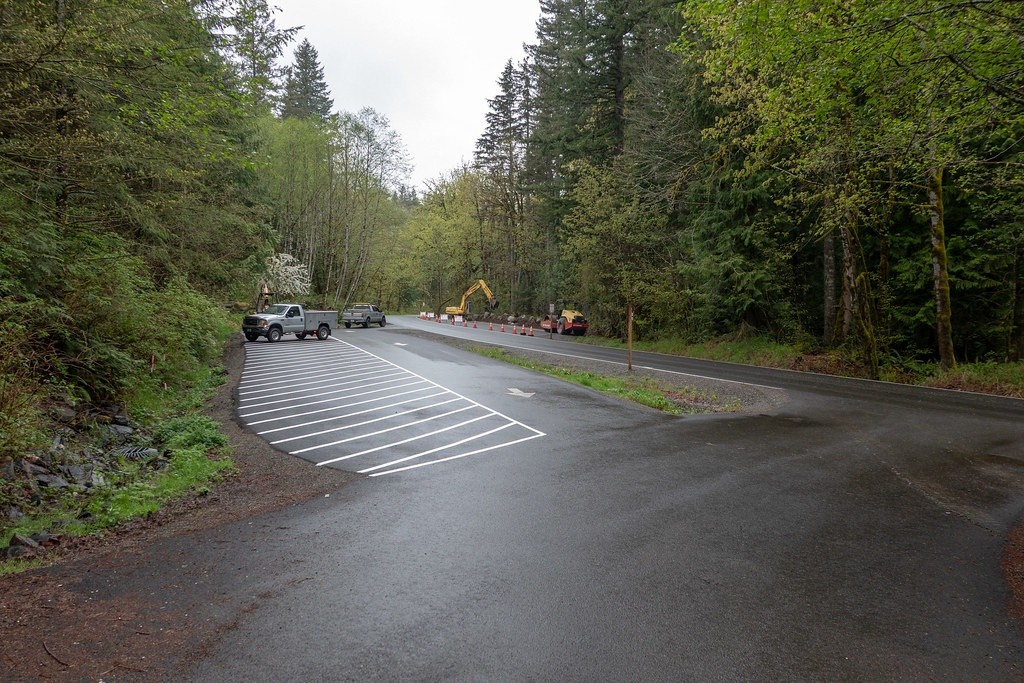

[540,309,590,336]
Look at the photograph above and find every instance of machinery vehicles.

[445,277,499,320]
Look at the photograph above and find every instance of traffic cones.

[450,318,455,325]
[488,323,493,330]
[438,317,442,323]
[512,324,517,334]
[435,316,438,322]
[499,323,505,332]
[473,321,477,328]
[463,320,468,327]
[528,326,534,336]
[519,323,526,335]
[419,314,431,321]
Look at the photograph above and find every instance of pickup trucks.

[341,303,386,329]
[241,303,339,342]
